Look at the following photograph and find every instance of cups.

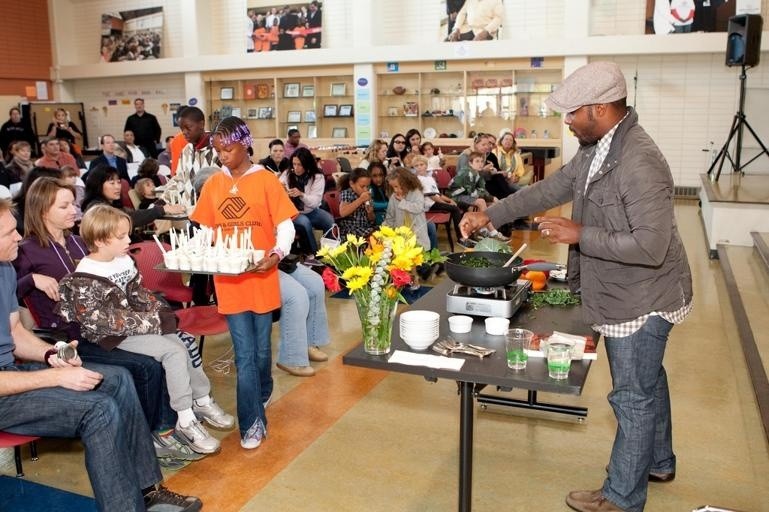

[448,315,473,335]
[484,316,510,337]
[503,326,535,371]
[162,244,265,275]
[362,198,372,209]
[545,342,573,379]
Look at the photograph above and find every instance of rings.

[547,230,550,236]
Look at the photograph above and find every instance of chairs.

[0,149,459,477]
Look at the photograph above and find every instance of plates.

[423,127,436,139]
[549,269,568,283]
[498,128,513,139]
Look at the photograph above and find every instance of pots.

[442,249,568,288]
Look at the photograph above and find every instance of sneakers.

[566,490,628,512]
[150,395,263,467]
[303,257,324,267]
[457,230,512,248]
[144,484,202,512]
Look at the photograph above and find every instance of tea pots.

[393,85,406,95]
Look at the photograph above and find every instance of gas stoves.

[445,278,538,319]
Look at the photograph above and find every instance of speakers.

[725,13,763,67]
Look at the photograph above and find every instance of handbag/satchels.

[278,254,301,273]
[319,223,341,251]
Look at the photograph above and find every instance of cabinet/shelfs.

[373,57,565,139]
[201,65,355,139]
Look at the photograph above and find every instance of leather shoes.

[308,346,328,361]
[276,362,316,377]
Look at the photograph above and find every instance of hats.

[545,61,627,113]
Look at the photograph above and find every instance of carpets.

[0,474,100,512]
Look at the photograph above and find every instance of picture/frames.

[220,82,353,138]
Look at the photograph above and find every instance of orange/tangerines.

[518,270,546,290]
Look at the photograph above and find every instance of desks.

[342,275,602,512]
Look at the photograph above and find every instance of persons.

[100,29,160,62]
[444,0,504,41]
[459,60,693,512]
[0,98,529,511]
[653,0,721,34]
[247,1,322,52]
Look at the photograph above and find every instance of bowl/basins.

[399,310,440,350]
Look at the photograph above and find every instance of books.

[522,334,597,361]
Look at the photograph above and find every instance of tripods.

[708,66,769,181]
